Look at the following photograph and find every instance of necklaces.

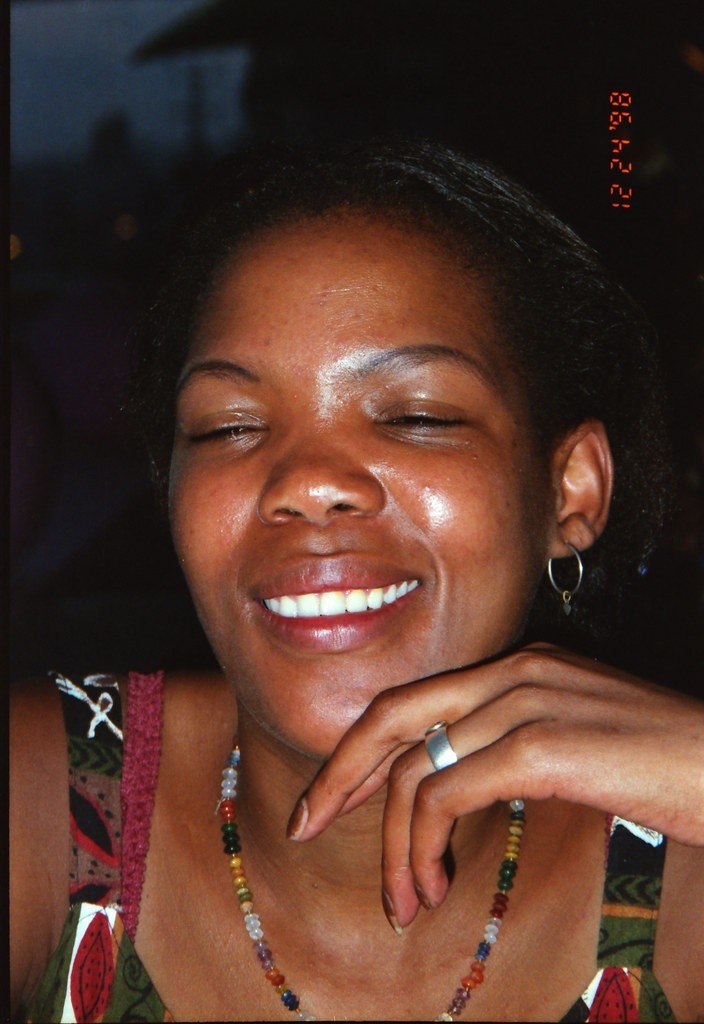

[212,734,525,1022]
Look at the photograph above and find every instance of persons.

[7,141,704,1022]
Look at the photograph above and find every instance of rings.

[424,720,459,771]
